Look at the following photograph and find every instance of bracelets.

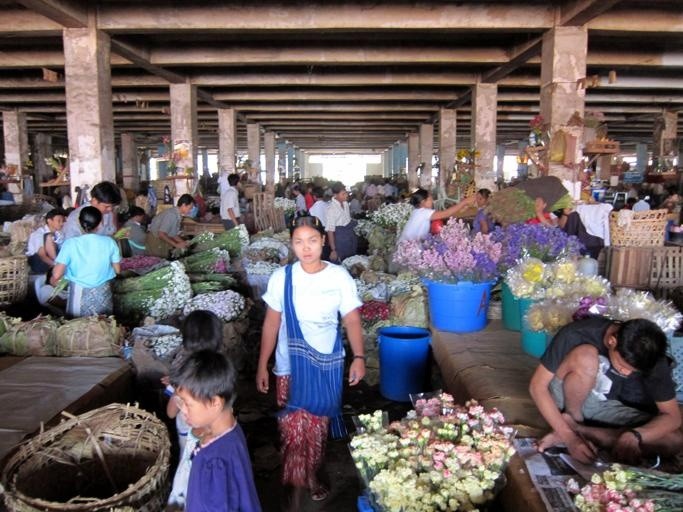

[352,355,365,360]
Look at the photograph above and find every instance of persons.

[276,180,399,201]
[61,182,121,247]
[350,192,369,219]
[624,198,636,210]
[124,207,147,256]
[526,196,571,227]
[634,192,650,210]
[398,190,476,240]
[146,194,194,257]
[27,210,64,272]
[326,182,357,264]
[176,351,261,512]
[50,206,121,319]
[135,191,151,218]
[305,183,315,215]
[220,174,241,231]
[168,310,223,504]
[529,318,682,463]
[294,187,307,217]
[472,189,495,234]
[257,217,365,509]
[309,187,328,261]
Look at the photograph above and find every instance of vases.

[519,299,558,361]
[251,282,268,302]
[169,169,174,176]
[461,176,467,182]
[62,176,70,181]
[500,280,539,333]
[535,134,542,145]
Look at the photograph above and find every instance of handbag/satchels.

[277,265,350,438]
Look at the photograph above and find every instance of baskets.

[152,179,174,200]
[448,183,475,200]
[0,255,28,305]
[606,247,683,287]
[53,187,72,197]
[245,185,258,200]
[609,208,667,246]
[5,403,171,512]
[22,194,56,215]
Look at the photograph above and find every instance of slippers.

[310,486,327,500]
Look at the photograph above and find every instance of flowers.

[494,220,586,271]
[250,228,276,241]
[193,223,251,256]
[606,286,681,338]
[190,283,224,294]
[340,254,370,279]
[118,254,168,273]
[164,150,181,168]
[171,231,214,258]
[272,196,296,216]
[273,229,291,249]
[458,148,480,164]
[460,165,466,171]
[502,254,546,297]
[162,133,170,159]
[573,297,606,319]
[45,148,70,173]
[245,238,290,283]
[183,288,246,323]
[355,300,389,329]
[112,259,192,320]
[134,330,183,359]
[530,115,548,133]
[396,219,501,284]
[372,256,402,276]
[548,259,578,293]
[580,275,611,299]
[190,274,239,286]
[58,314,125,357]
[350,387,517,512]
[523,304,544,331]
[543,292,584,333]
[177,246,232,273]
[49,226,133,304]
[570,461,683,512]
[354,200,415,256]
[354,278,394,303]
[32,317,60,358]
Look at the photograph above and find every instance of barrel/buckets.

[503,281,521,331]
[520,297,547,358]
[375,326,431,402]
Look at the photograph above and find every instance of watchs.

[627,429,642,446]
